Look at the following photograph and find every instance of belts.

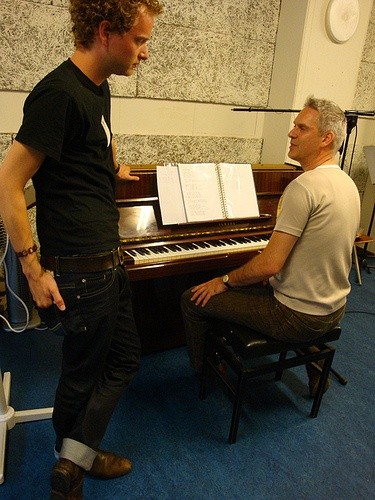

[40,246,124,273]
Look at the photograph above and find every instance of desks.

[353,231,374,286]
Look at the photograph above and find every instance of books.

[155,163,260,224]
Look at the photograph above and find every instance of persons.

[178,96,361,380]
[0,0,164,500]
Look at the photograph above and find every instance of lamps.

[326,0,361,45]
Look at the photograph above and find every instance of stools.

[196,317,337,444]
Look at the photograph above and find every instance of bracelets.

[16,245,37,257]
[114,164,120,174]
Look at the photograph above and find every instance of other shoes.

[81,449,132,480]
[52,458,84,500]
[308,349,330,398]
[191,360,224,375]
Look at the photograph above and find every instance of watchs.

[223,274,233,289]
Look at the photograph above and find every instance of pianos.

[116,162,304,357]
